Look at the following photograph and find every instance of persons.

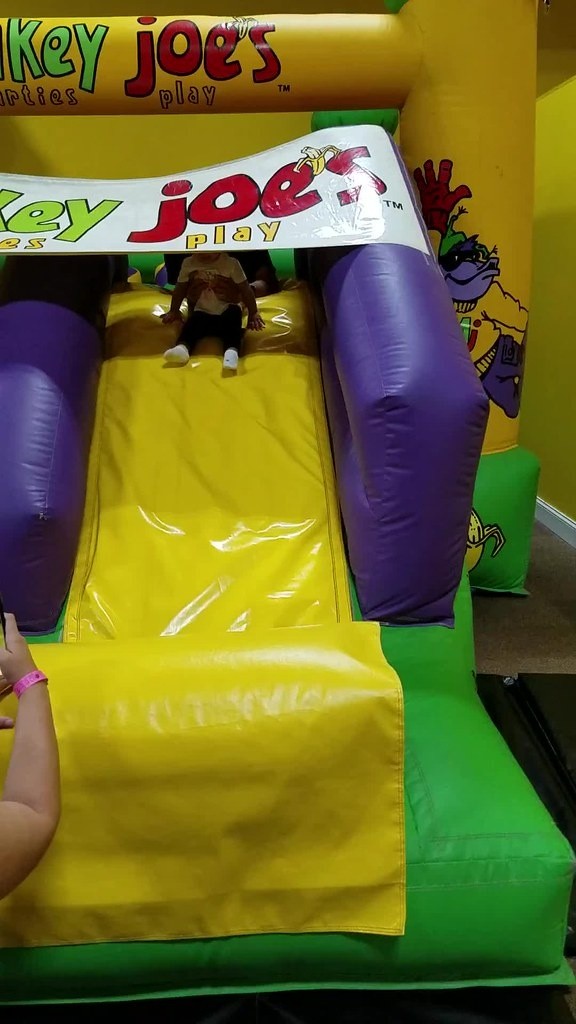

[0,613,61,899]
[160,250,279,370]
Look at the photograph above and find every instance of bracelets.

[14,671,49,698]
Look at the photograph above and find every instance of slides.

[0,277,575,1019]
